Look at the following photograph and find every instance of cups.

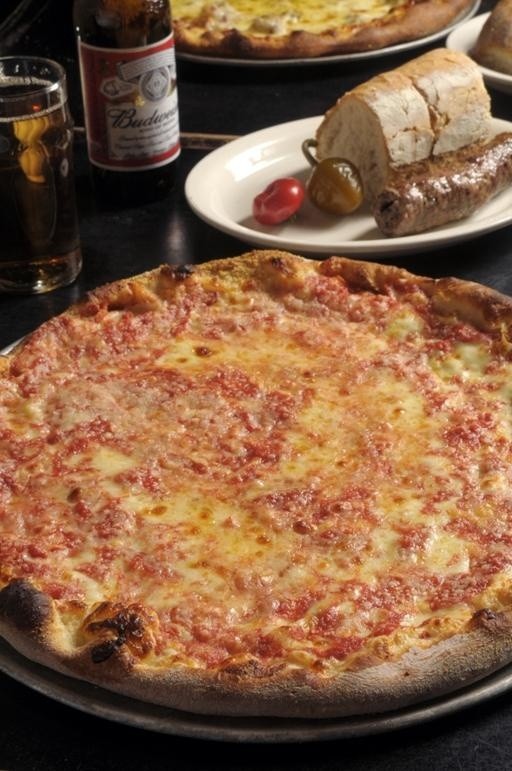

[0,54,85,295]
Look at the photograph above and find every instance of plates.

[446,11,510,88]
[174,2,484,70]
[184,113,510,260]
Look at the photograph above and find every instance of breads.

[470,0,512,75]
[314,47,492,201]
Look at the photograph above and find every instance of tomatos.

[252,177,305,226]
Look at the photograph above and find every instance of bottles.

[77,0,183,207]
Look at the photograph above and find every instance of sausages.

[373,132,512,238]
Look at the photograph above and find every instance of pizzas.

[169,0,471,60]
[0,248,512,718]
